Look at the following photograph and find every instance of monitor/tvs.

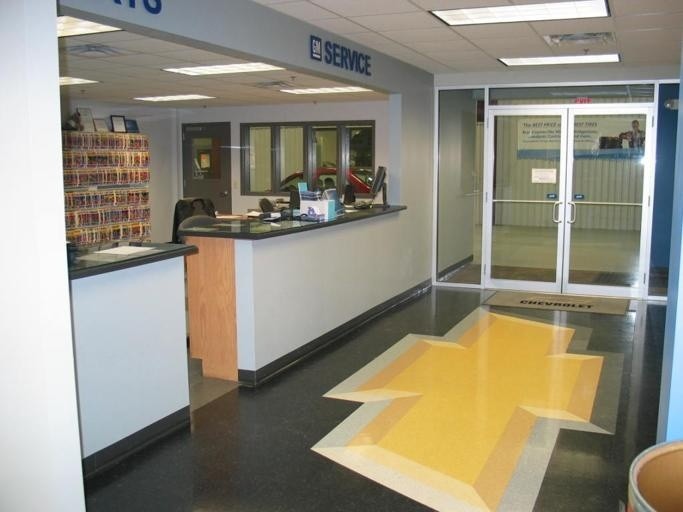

[370,165,387,195]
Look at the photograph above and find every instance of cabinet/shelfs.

[63,132,150,247]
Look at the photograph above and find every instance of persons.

[322,178,337,191]
[620,120,647,148]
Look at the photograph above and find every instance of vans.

[350,128,372,167]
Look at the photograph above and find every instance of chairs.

[172,199,213,241]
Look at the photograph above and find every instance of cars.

[280,166,375,194]
